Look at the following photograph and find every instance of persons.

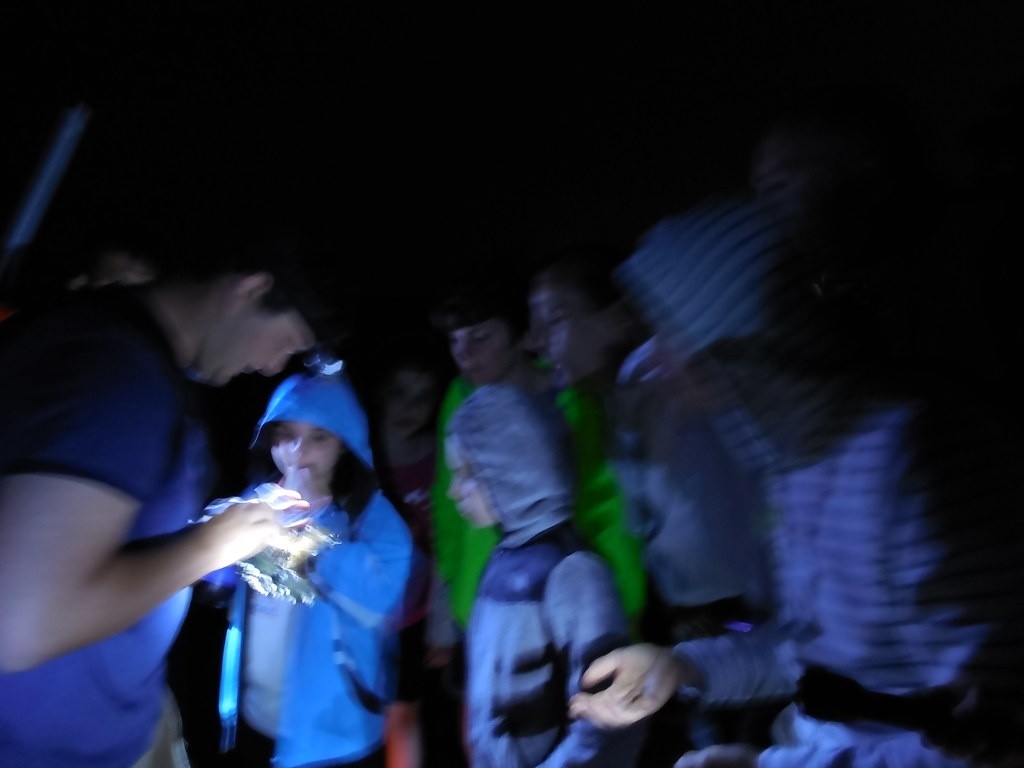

[200,373,414,768]
[0,229,354,768]
[93,283,811,768]
[444,380,624,768]
[568,210,992,768]
